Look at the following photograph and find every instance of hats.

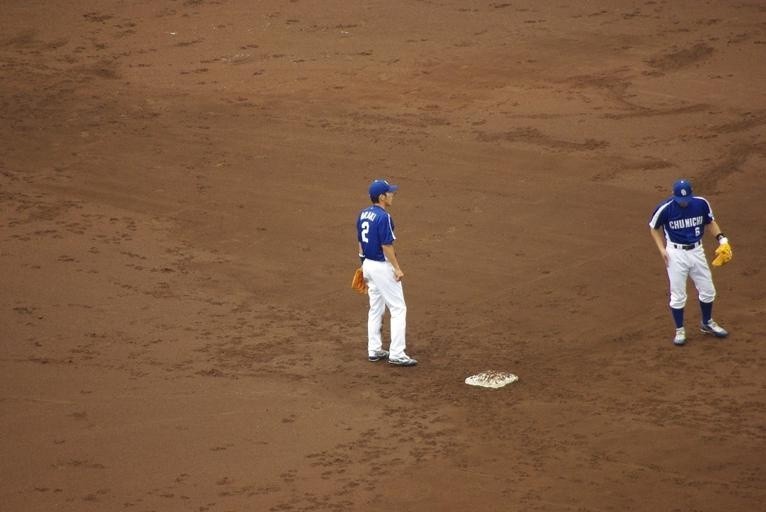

[367,179,400,193]
[671,180,694,202]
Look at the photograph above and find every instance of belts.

[674,239,701,250]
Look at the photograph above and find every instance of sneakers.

[697,317,729,337]
[673,326,687,345]
[367,350,418,366]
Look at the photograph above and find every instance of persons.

[649,180,733,346]
[356,180,419,367]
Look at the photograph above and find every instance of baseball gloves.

[352,269,368,295]
[711,243,731,268]
